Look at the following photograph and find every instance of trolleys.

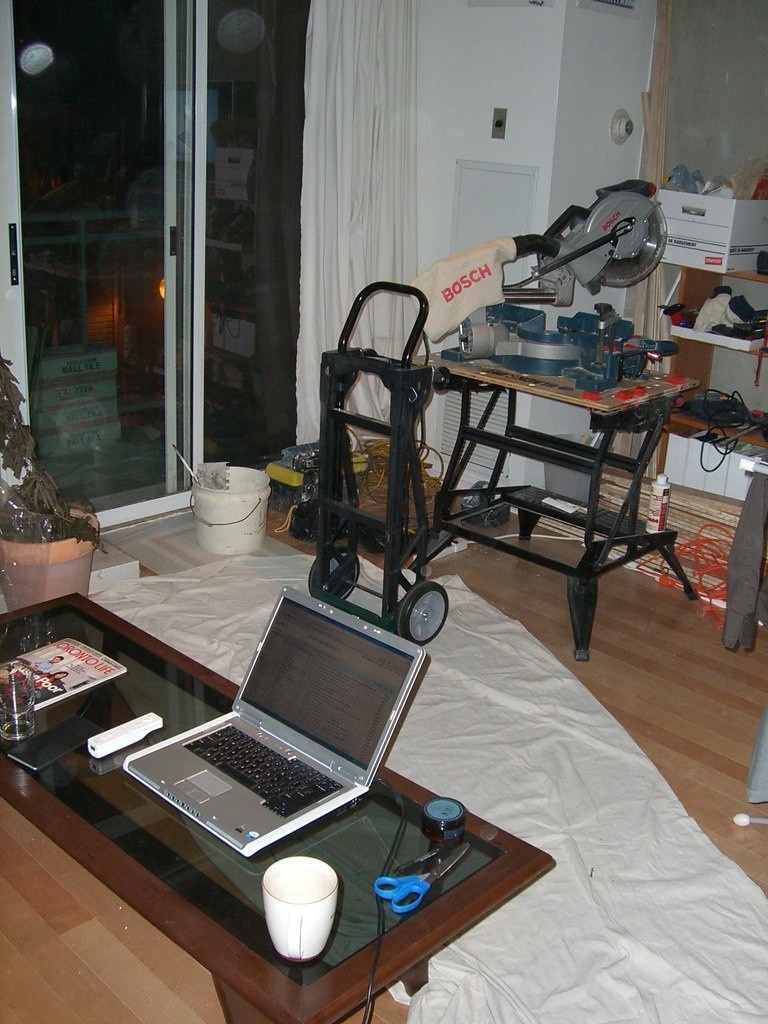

[308,282,450,647]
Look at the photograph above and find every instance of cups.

[262,857,337,962]
[0,661,34,741]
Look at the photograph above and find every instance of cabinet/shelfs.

[132,81,304,407]
[656,266,768,474]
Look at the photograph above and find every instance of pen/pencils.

[393,848,439,873]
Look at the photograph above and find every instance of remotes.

[87,712,163,759]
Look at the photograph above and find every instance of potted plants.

[0,351,109,613]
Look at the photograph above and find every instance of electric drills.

[673,399,748,421]
[264,442,321,471]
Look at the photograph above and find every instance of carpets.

[95,511,305,576]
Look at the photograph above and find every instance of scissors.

[373,842,470,913]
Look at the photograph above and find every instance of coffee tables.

[0,591,557,1024]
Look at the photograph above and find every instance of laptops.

[124,586,426,858]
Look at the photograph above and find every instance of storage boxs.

[215,147,255,201]
[88,540,141,595]
[30,343,122,460]
[212,317,256,360]
[658,189,768,274]
[266,453,368,516]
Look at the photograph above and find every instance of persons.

[30,655,63,673]
[33,671,69,692]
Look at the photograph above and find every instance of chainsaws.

[408,178,680,393]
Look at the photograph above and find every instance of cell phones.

[6,715,104,771]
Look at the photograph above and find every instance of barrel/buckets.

[190,467,270,554]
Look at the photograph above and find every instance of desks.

[407,349,700,662]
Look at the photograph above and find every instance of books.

[0,637,127,718]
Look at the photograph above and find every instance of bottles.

[645,474,671,535]
